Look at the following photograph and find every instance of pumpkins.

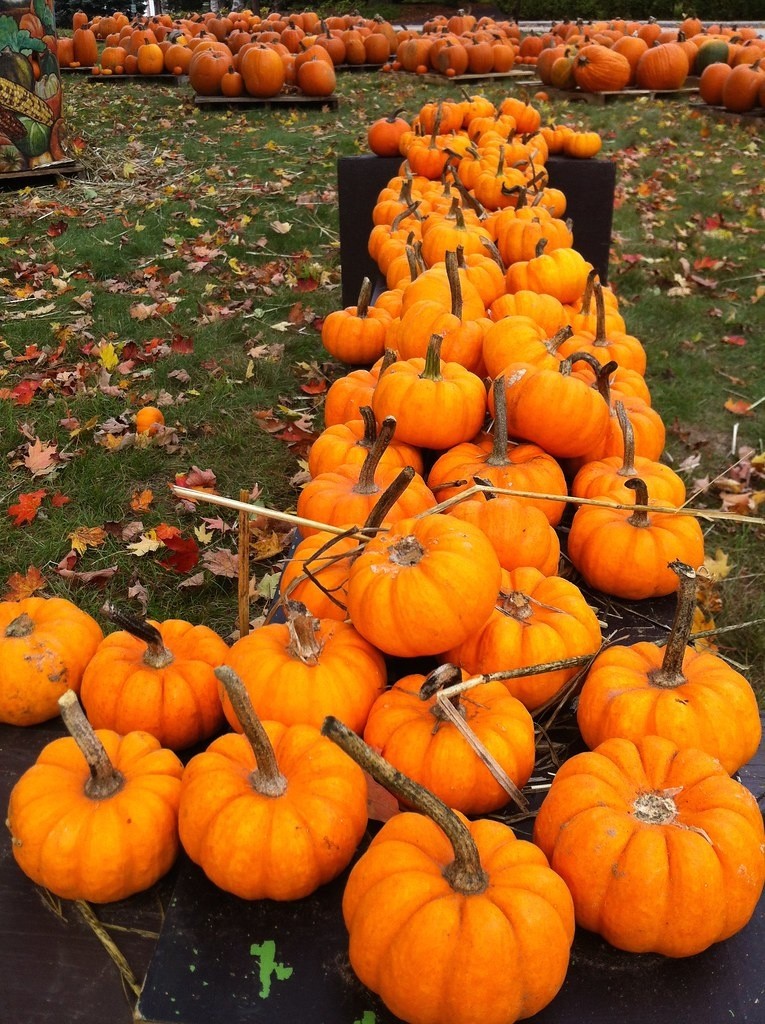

[0,93,765,1024]
[57,10,765,113]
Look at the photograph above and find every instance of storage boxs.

[336,153,616,305]
